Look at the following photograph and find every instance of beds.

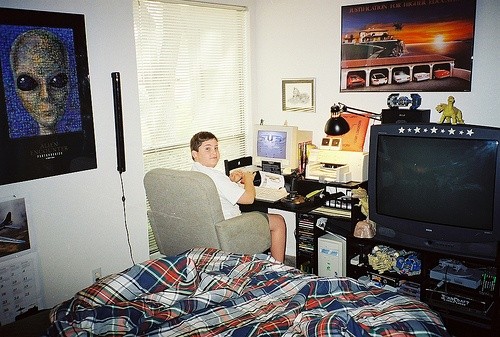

[0,248,447,337]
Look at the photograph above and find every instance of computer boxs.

[318,234,347,278]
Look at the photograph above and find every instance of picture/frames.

[280,77,316,113]
[339,0,477,92]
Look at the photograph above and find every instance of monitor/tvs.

[368,122,500,263]
[253,124,312,175]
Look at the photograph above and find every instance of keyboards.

[237,183,289,202]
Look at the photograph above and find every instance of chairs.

[143,169,272,257]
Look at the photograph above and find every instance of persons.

[190,131,286,263]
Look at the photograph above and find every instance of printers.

[306,148,368,183]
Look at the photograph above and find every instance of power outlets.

[92,268,101,283]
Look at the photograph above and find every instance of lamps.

[325,102,382,135]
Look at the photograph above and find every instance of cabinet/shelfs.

[224,156,500,337]
